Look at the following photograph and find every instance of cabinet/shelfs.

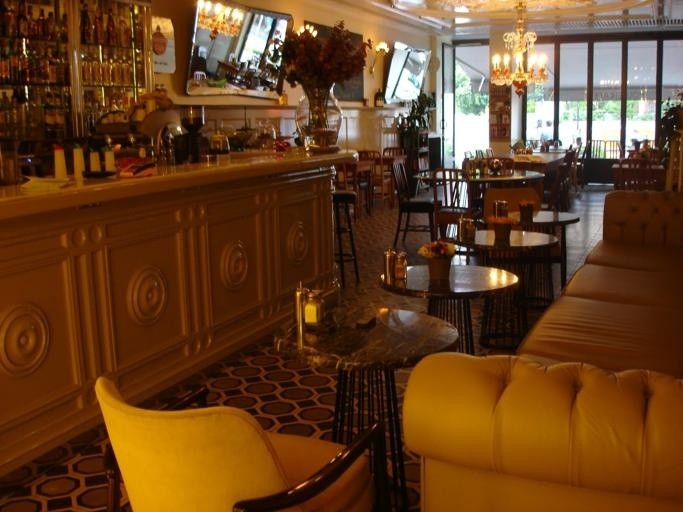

[76,0,156,136]
[401,127,430,195]
[0,0,76,140]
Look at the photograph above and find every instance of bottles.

[375,87,384,107]
[302,290,325,324]
[53,142,115,181]
[0,0,139,46]
[0,36,145,87]
[384,248,396,282]
[461,155,476,177]
[0,83,132,137]
[395,251,408,282]
[295,280,307,324]
[458,215,466,241]
[279,89,288,105]
[467,218,475,240]
[499,200,509,218]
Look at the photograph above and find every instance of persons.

[566,136,582,153]
[531,119,543,141]
[539,120,562,146]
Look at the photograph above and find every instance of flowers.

[656,93,683,150]
[265,23,372,87]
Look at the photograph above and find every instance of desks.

[273,139,592,512]
[377,263,520,365]
[273,307,460,507]
[475,211,581,289]
[447,228,560,359]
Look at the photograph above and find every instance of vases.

[301,83,329,136]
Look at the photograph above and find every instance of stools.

[332,190,362,289]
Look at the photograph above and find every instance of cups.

[363,98,370,106]
[145,143,176,167]
[258,127,278,153]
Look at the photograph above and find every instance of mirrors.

[185,0,294,99]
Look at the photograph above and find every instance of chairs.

[194,53,260,89]
[92,378,379,512]
[612,150,664,190]
[331,139,592,247]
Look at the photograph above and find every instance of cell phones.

[356,316,376,327]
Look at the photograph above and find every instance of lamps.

[196,0,250,40]
[491,0,549,95]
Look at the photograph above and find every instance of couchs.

[401,189,683,512]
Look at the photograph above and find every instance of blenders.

[178,103,209,154]
